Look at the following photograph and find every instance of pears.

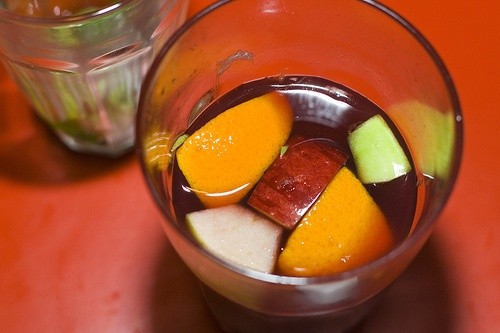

[185,203,284,273]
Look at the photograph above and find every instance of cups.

[130,0,466,319]
[1,0,188,157]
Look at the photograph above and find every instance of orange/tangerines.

[176,90,294,208]
[276,166,396,277]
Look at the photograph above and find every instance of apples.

[247,132,349,229]
